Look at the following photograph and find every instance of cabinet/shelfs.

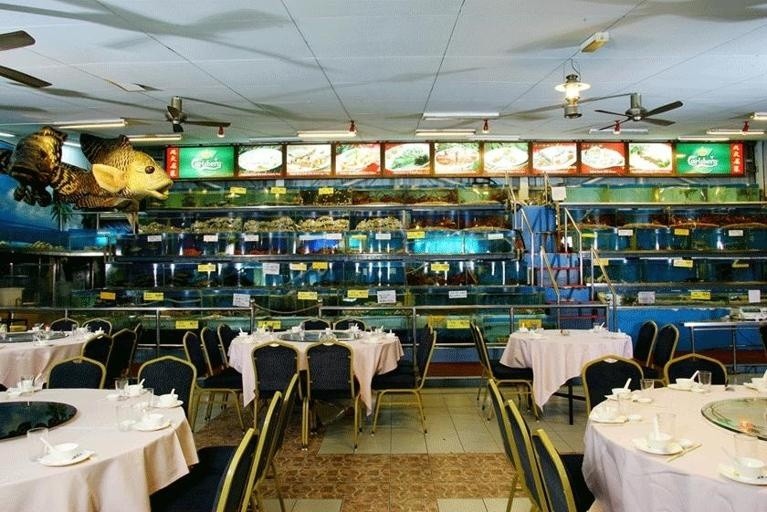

[556,199,767,289]
[99,202,542,297]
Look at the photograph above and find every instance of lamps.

[590,127,648,135]
[416,128,476,137]
[677,112,766,143]
[349,120,356,137]
[127,133,182,142]
[483,120,490,134]
[53,118,129,130]
[556,58,590,118]
[216,126,224,138]
[422,111,500,122]
[297,130,358,138]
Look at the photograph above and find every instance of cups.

[0,323,91,347]
[588,370,767,486]
[640,372,758,459]
[20,376,183,463]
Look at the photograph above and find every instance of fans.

[121,97,231,134]
[596,93,683,131]
[0,30,53,88]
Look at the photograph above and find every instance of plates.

[236,148,284,172]
[45,399,183,466]
[534,146,577,171]
[581,148,626,169]
[435,145,478,172]
[385,143,430,172]
[486,147,528,167]
